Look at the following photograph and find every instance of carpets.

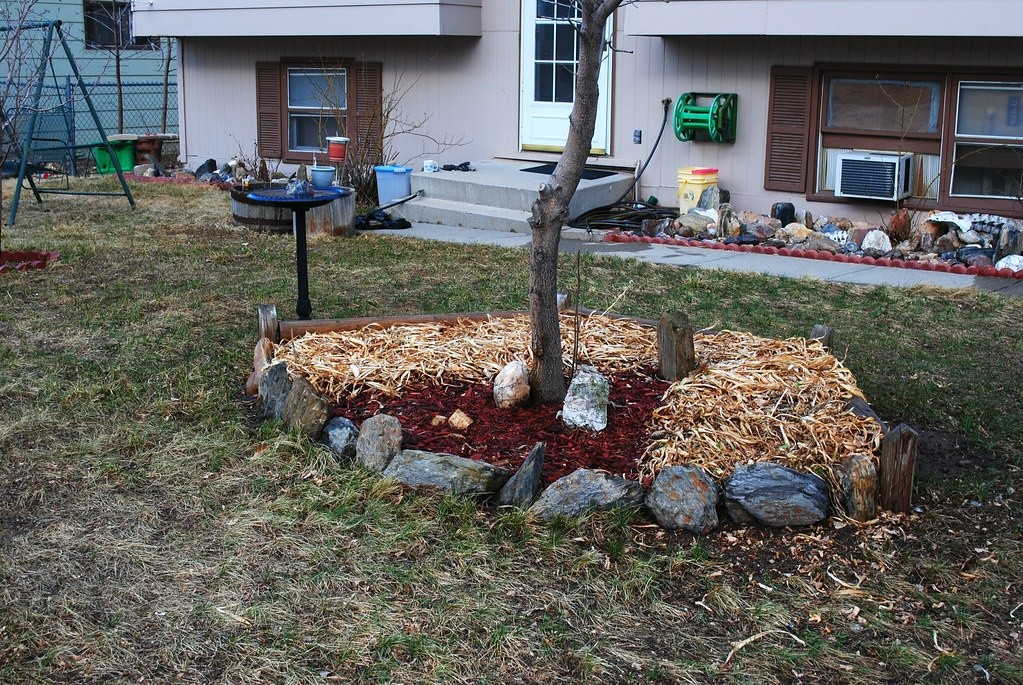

[519,164,619,180]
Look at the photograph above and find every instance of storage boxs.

[94,140,136,173]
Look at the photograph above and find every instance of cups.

[424,160,439,173]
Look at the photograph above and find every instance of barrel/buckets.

[374,165,412,219]
[676,168,718,216]
[311,166,336,187]
[326,136,350,162]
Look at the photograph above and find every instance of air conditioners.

[835,151,913,201]
